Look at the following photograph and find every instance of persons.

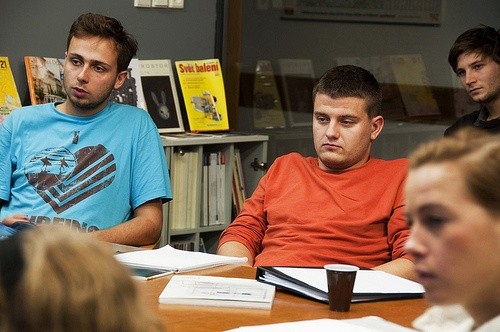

[0,221,141,332]
[444,25,500,137]
[218,64,419,281]
[404,129,500,332]
[0,14,173,245]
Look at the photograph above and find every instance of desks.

[105,243,433,332]
[264,121,456,167]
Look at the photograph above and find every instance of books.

[111,60,144,110]
[338,56,382,82]
[451,68,480,117]
[170,149,246,230]
[159,275,276,309]
[253,59,285,129]
[390,55,440,117]
[278,58,316,128]
[117,239,249,281]
[255,265,426,304]
[0,57,22,121]
[23,55,67,105]
[136,59,184,133]
[175,59,229,131]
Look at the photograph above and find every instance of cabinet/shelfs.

[157,130,268,254]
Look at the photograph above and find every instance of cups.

[324,264,360,312]
